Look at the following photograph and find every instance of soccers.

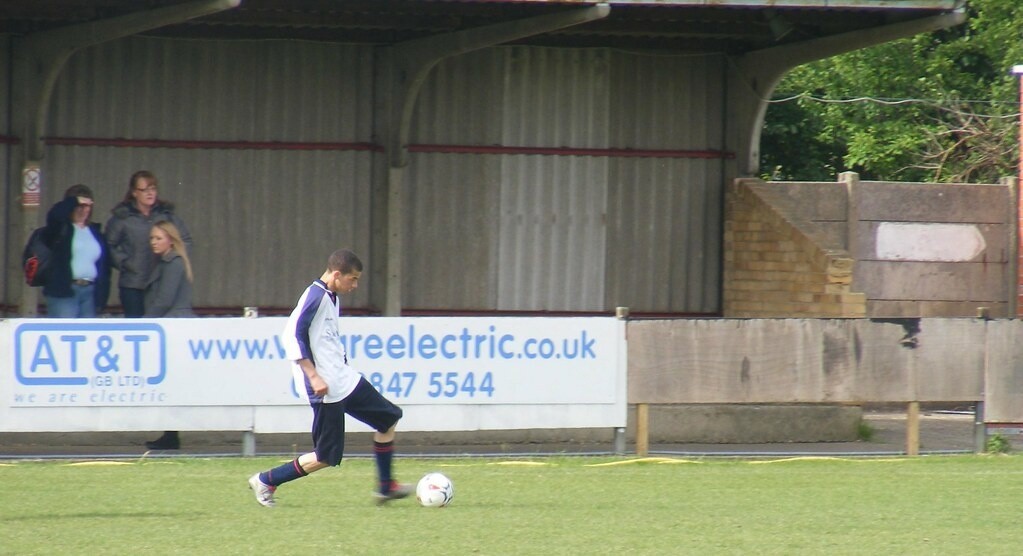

[414,472,455,509]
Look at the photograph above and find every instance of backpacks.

[22,225,67,287]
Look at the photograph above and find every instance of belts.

[71,278,93,286]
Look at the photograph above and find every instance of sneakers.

[371,480,413,501]
[248,471,277,507]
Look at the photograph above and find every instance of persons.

[20,169,200,450]
[245,247,412,509]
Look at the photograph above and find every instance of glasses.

[135,187,158,193]
